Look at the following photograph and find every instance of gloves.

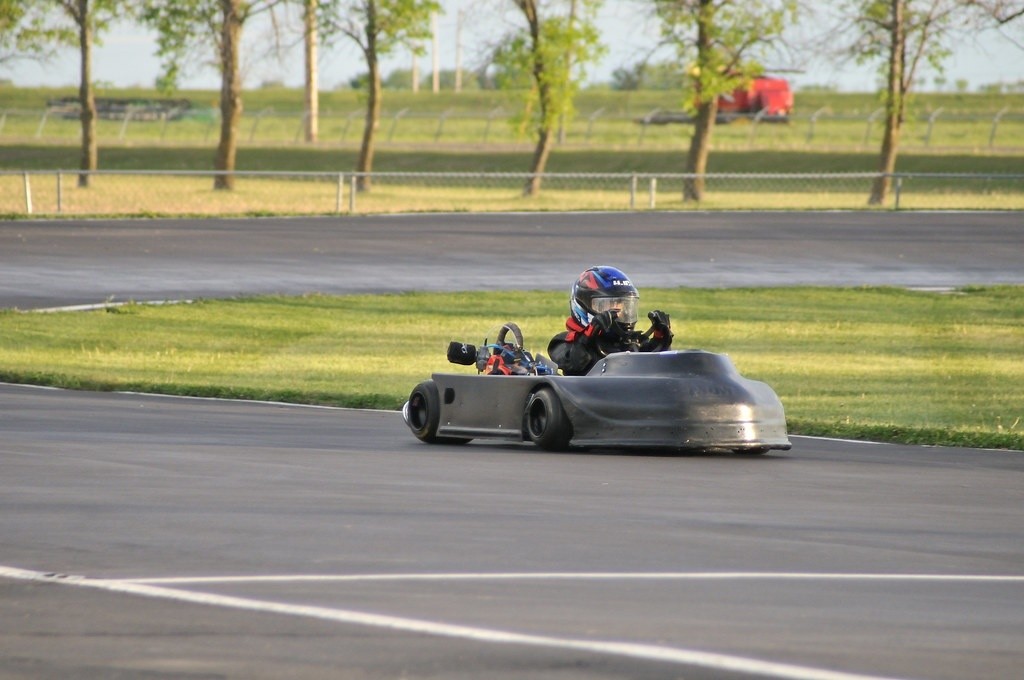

[648,310,674,339]
[580,308,620,347]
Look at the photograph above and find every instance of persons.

[547,266,674,377]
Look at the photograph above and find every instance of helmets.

[569,266,639,331]
